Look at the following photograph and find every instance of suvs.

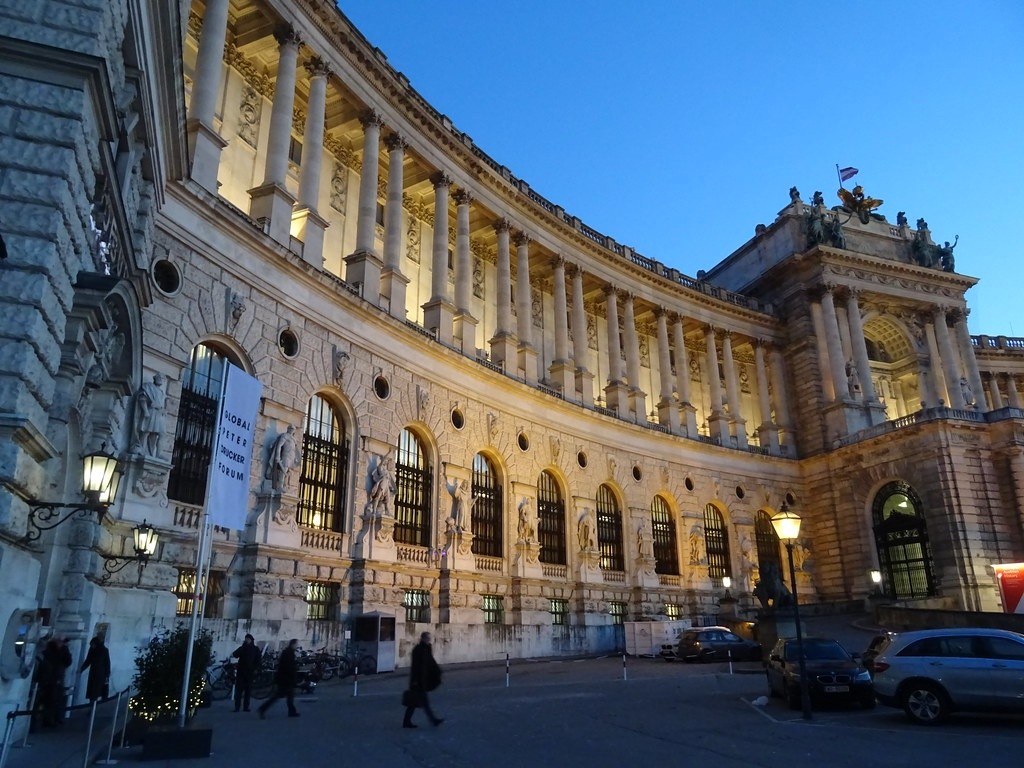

[659,626,731,661]
[768,639,873,711]
[874,628,1024,721]
[679,630,758,663]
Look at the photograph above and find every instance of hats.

[245,634,254,641]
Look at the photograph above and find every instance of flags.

[206,361,265,531]
[839,166,859,181]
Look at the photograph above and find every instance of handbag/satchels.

[402,690,427,708]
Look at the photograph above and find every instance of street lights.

[769,503,814,721]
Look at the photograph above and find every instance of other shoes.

[403,722,418,728]
[243,709,251,712]
[433,718,446,727]
[288,713,301,718]
[232,709,239,712]
[256,710,266,720]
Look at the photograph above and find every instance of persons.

[29,635,74,734]
[578,507,598,551]
[809,196,960,274]
[266,422,301,495]
[960,374,975,405]
[135,371,169,461]
[843,356,860,392]
[402,632,444,729]
[369,446,398,518]
[257,638,302,721]
[518,497,540,545]
[78,636,112,717]
[639,517,757,568]
[228,633,264,713]
[452,476,479,533]
